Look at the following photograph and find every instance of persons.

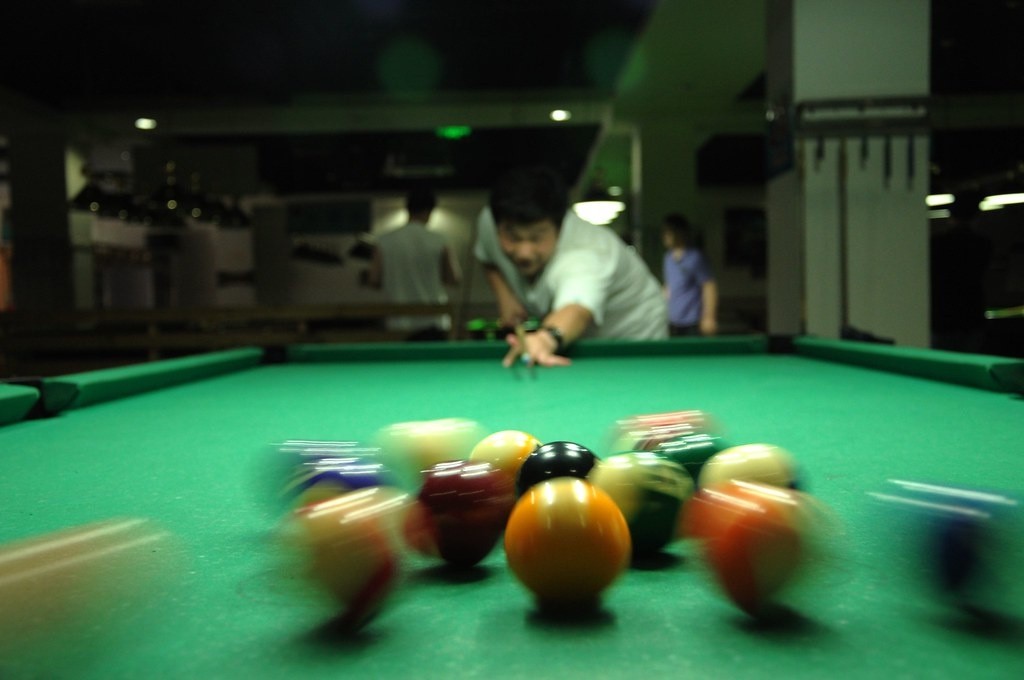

[472,163,670,364]
[359,178,464,342]
[659,214,719,335]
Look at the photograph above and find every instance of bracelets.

[540,325,562,353]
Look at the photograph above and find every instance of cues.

[517,315,533,364]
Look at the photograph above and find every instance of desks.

[0,336,1024,680]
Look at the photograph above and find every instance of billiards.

[502,476,630,602]
[515,441,596,493]
[0,408,1024,634]
